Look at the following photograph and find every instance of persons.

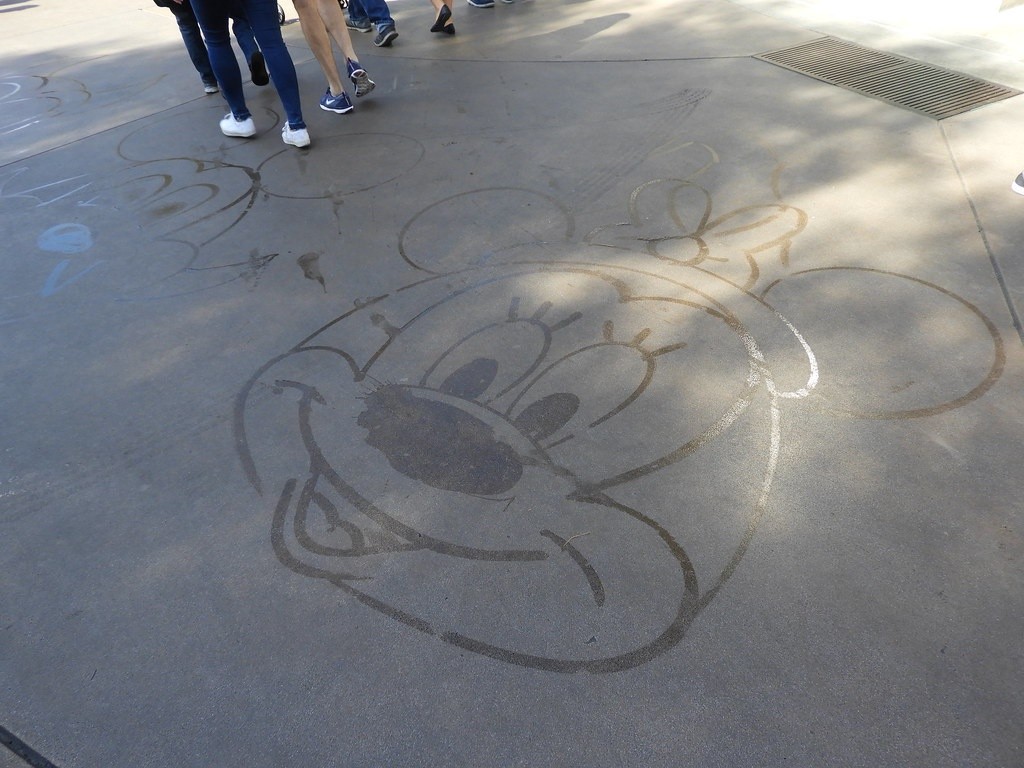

[345,0,399,47]
[429,0,457,34]
[292,0,376,114]
[151,0,311,149]
[466,0,515,9]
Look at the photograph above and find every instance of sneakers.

[373,25,398,46]
[345,16,371,33]
[219,112,256,137]
[319,86,354,114]
[282,120,311,147]
[346,57,376,98]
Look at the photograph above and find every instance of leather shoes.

[430,3,455,34]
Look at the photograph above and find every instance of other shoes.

[202,79,218,93]
[467,0,495,8]
[251,51,269,86]
